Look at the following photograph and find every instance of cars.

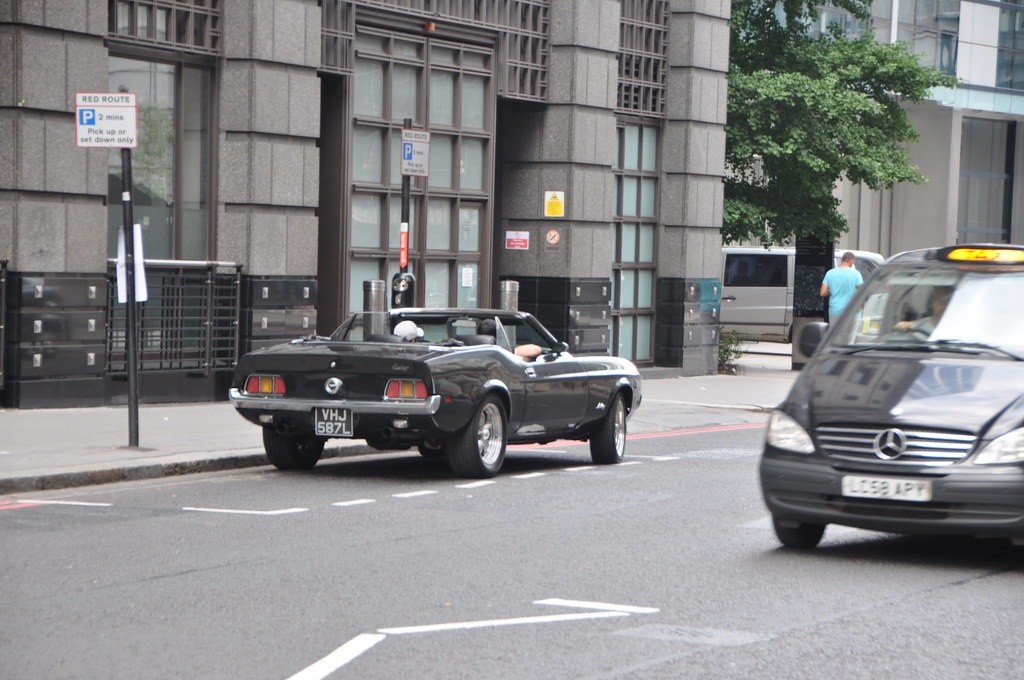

[229,305,645,483]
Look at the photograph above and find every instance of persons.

[818,251,867,345]
[393,321,425,343]
[477,317,544,362]
[894,285,973,345]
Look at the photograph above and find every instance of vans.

[721,246,889,341]
[759,242,1024,564]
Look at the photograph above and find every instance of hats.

[393,319,424,340]
